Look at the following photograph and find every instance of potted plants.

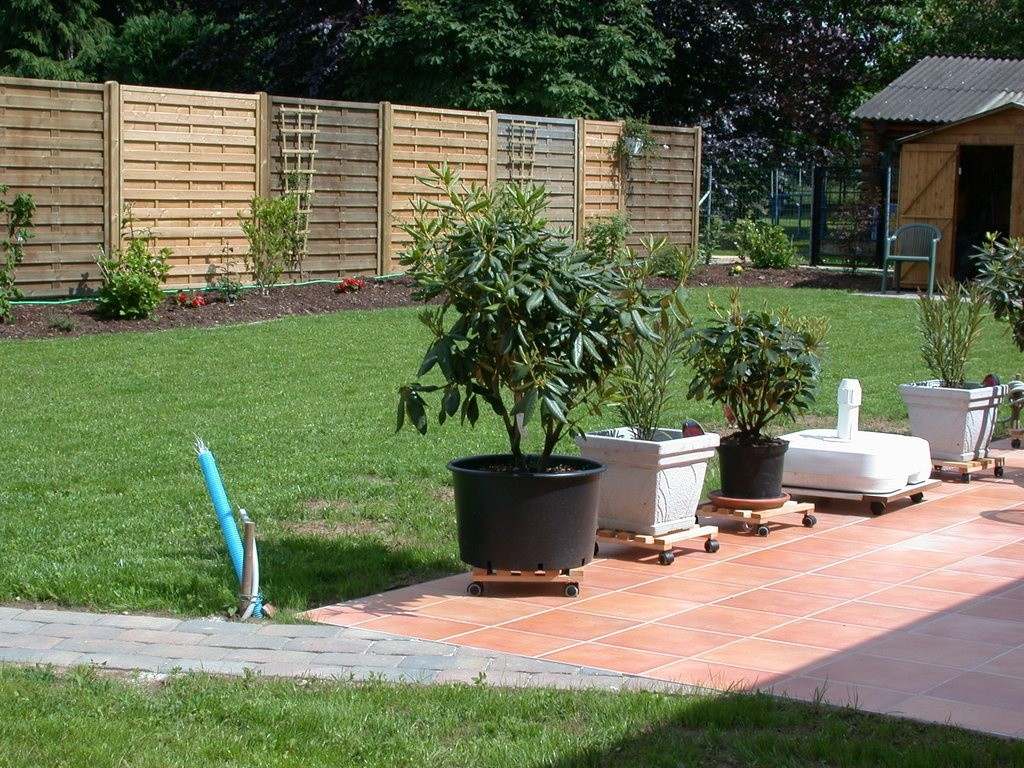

[386,161,834,572]
[609,111,666,185]
[897,276,1009,463]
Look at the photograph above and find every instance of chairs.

[880,223,942,297]
